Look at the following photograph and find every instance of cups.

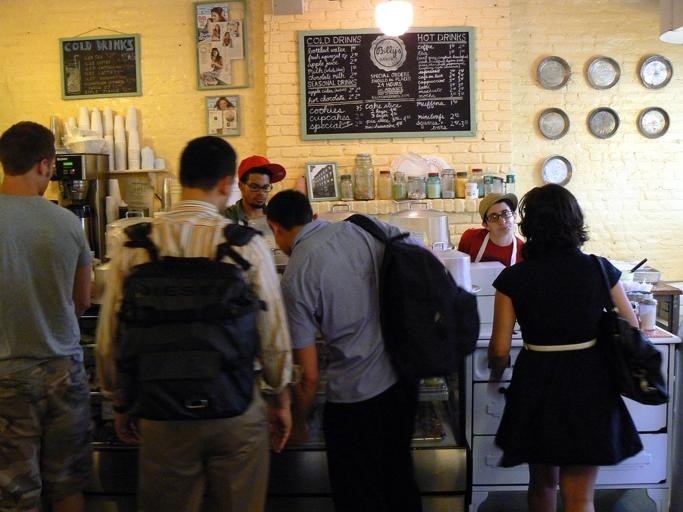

[50,104,167,168]
[103,176,122,225]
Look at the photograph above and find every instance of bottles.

[336,152,516,201]
[637,292,656,333]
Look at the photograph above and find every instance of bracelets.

[111,403,129,415]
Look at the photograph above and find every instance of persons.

[0,121,95,509]
[458,193,524,267]
[486,185,643,512]
[214,98,236,110]
[195,4,240,88]
[222,154,290,255]
[263,189,433,512]
[95,134,293,508]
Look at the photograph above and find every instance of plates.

[638,56,672,88]
[586,57,620,88]
[536,108,569,139]
[586,106,619,138]
[536,55,570,89]
[638,107,671,138]
[541,154,572,187]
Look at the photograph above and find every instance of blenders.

[33,152,112,274]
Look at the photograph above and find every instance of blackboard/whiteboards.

[60,34,141,99]
[298,26,476,140]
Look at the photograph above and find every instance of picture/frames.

[304,160,340,203]
[195,2,252,140]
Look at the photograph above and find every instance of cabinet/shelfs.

[460,326,683,510]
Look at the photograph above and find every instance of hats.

[478,192,517,220]
[237,156,285,183]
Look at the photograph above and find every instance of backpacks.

[343,214,479,444]
[112,222,267,421]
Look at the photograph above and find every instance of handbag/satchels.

[601,313,668,405]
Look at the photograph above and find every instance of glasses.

[484,210,516,221]
[245,181,272,193]
